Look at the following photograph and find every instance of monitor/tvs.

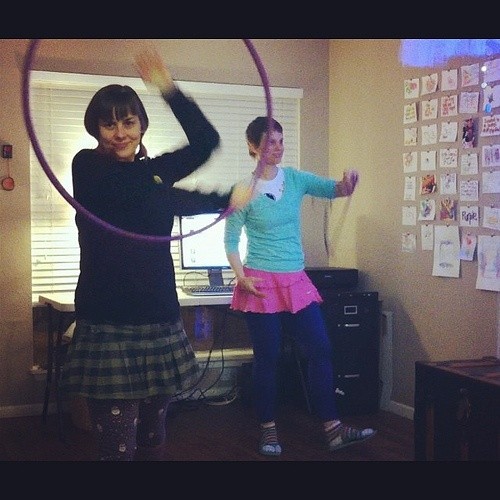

[178,209,251,285]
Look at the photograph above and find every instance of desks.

[40,288,256,434]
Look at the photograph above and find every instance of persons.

[54,49,252,459]
[224,116,376,457]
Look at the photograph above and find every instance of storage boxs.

[413,357,500,461]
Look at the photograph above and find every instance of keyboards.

[182,286,236,296]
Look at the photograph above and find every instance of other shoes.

[326,426,377,451]
[259,439,282,455]
